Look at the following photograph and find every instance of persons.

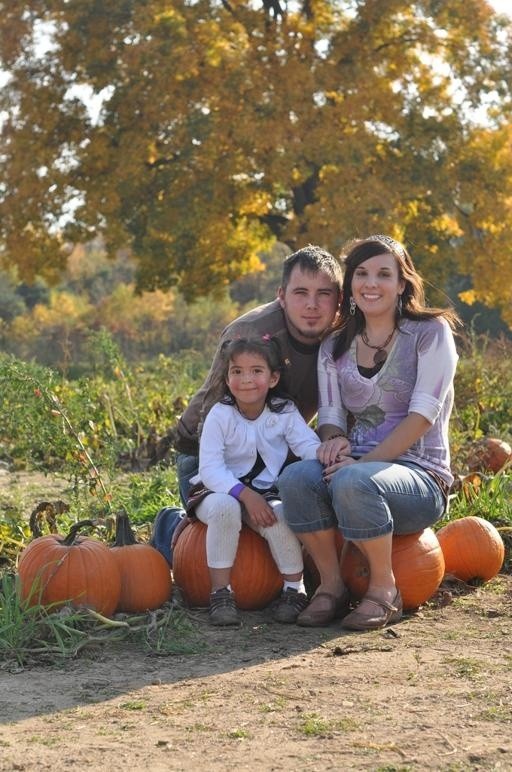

[153,243,342,572]
[185,336,306,627]
[277,234,457,630]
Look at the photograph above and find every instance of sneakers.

[276,588,309,623]
[209,585,240,625]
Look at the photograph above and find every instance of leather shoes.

[341,590,403,630]
[297,587,351,626]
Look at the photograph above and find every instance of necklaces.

[361,330,398,364]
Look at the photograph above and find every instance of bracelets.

[327,433,352,442]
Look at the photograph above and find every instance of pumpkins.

[299,527,446,613]
[436,516,504,588]
[172,520,284,608]
[17,502,121,625]
[469,438,512,476]
[109,506,173,612]
